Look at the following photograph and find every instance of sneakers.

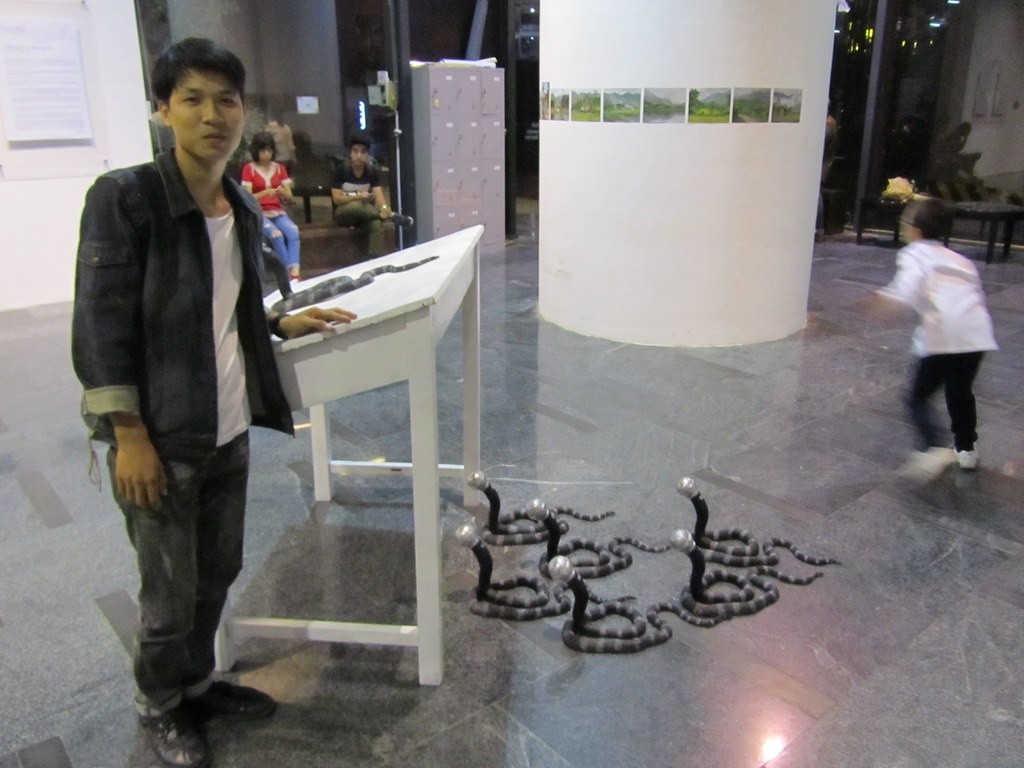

[903,448,955,476]
[392,212,414,229]
[954,444,979,469]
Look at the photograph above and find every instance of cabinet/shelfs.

[408,63,506,253]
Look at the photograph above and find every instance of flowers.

[881,175,919,205]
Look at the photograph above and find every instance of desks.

[220,222,491,691]
[929,198,1024,265]
[856,199,950,244]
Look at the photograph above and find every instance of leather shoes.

[184,681,276,722]
[136,703,210,768]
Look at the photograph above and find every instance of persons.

[940,123,980,203]
[331,138,413,260]
[266,110,297,176]
[886,112,926,188]
[876,200,1002,478]
[814,99,837,243]
[241,131,304,283]
[71,38,357,768]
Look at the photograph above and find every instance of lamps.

[514,23,539,61]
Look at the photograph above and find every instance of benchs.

[231,164,386,255]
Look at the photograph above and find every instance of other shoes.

[816,231,826,242]
[288,273,301,286]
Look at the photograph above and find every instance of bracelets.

[272,313,290,341]
[380,205,387,208]
